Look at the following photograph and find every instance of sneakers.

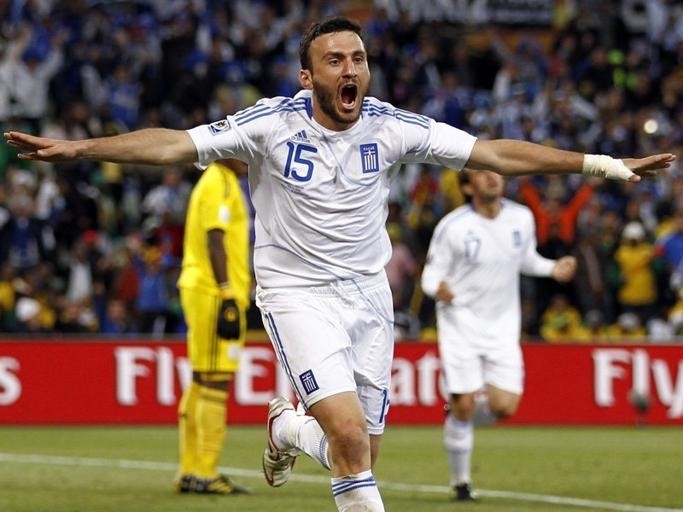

[263,395,298,488]
[454,483,476,501]
[176,473,249,496]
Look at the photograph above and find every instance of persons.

[3,15,677,511]
[175,149,255,494]
[0,0,683,335]
[421,160,579,501]
